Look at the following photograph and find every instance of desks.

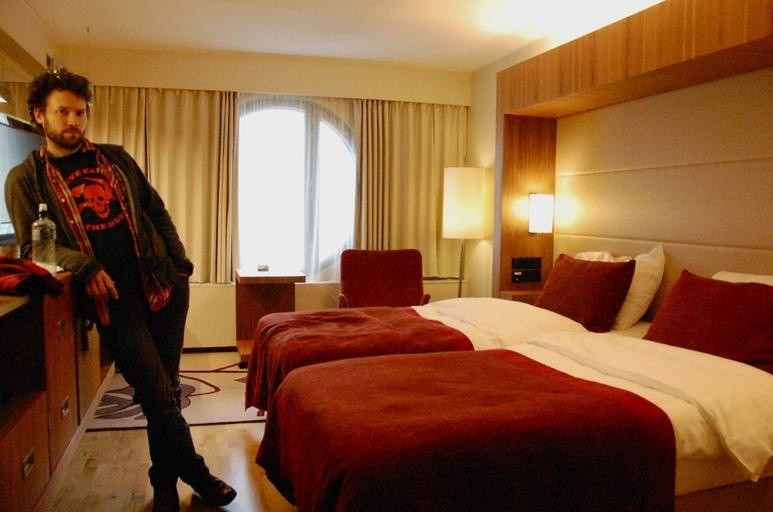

[235,268,305,369]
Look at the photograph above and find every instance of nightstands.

[500,290,540,304]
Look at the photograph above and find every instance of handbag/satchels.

[0,256,63,300]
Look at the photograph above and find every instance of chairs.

[338,249,431,308]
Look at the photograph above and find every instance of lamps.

[528,191,554,234]
[442,168,486,297]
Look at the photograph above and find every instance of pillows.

[642,268,773,375]
[712,271,773,286]
[575,245,666,331]
[536,254,636,333]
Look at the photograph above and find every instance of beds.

[255,333,773,512]
[246,297,650,410]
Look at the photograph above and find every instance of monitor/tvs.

[0,114,48,247]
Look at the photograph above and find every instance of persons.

[3,66,236,512]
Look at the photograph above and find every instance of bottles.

[30,202,57,280]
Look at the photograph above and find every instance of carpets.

[87,371,265,431]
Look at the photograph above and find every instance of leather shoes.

[152,485,180,511]
[185,470,238,507]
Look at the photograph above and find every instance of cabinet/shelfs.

[0,271,115,511]
